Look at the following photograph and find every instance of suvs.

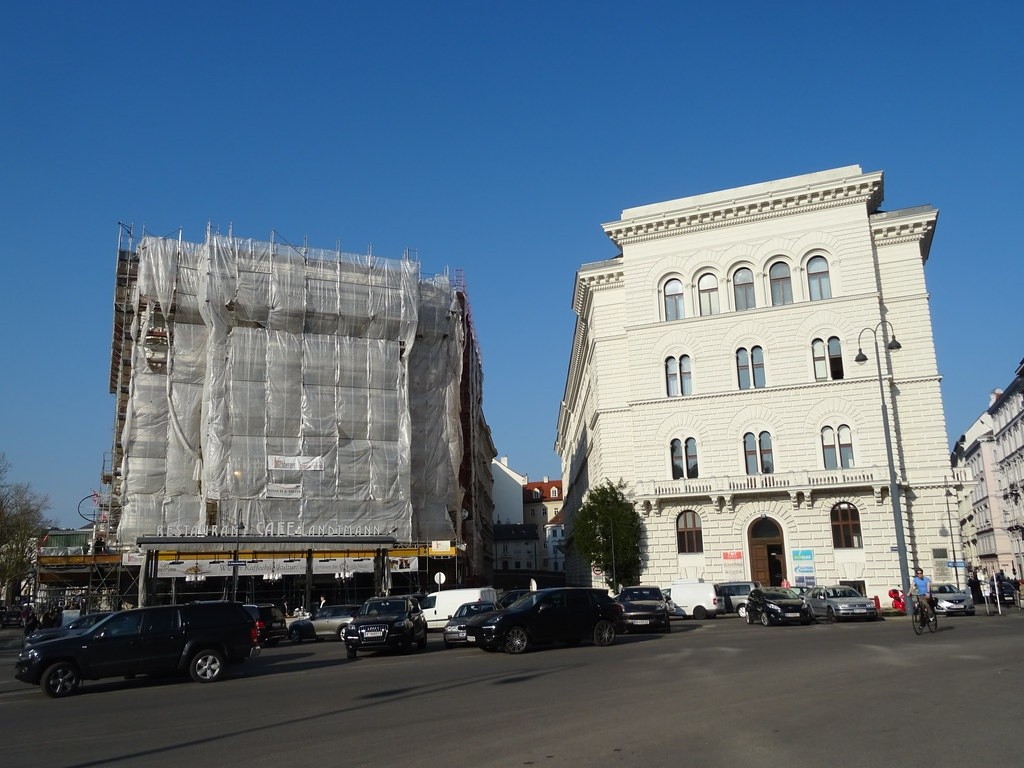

[242,602,289,646]
[804,585,878,623]
[344,595,428,659]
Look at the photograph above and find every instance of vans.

[417,587,498,630]
[717,581,765,619]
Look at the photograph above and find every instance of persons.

[906,568,934,619]
[24,607,62,635]
[1007,577,1020,591]
[281,600,290,616]
[320,596,328,608]
[96,537,105,554]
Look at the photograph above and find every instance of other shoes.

[928,617,935,623]
[919,624,926,629]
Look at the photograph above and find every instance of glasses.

[916,573,923,574]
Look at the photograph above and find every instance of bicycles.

[907,593,938,635]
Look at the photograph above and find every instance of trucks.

[660,582,726,620]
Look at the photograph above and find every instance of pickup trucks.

[13,600,258,699]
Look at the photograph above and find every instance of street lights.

[942,474,960,590]
[236,508,246,561]
[853,321,915,618]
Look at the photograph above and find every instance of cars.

[24,612,137,648]
[911,582,976,616]
[789,585,811,598]
[466,587,627,654]
[965,575,1022,605]
[745,586,814,625]
[443,590,530,648]
[284,605,380,645]
[616,585,672,634]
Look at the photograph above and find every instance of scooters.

[889,584,915,612]
